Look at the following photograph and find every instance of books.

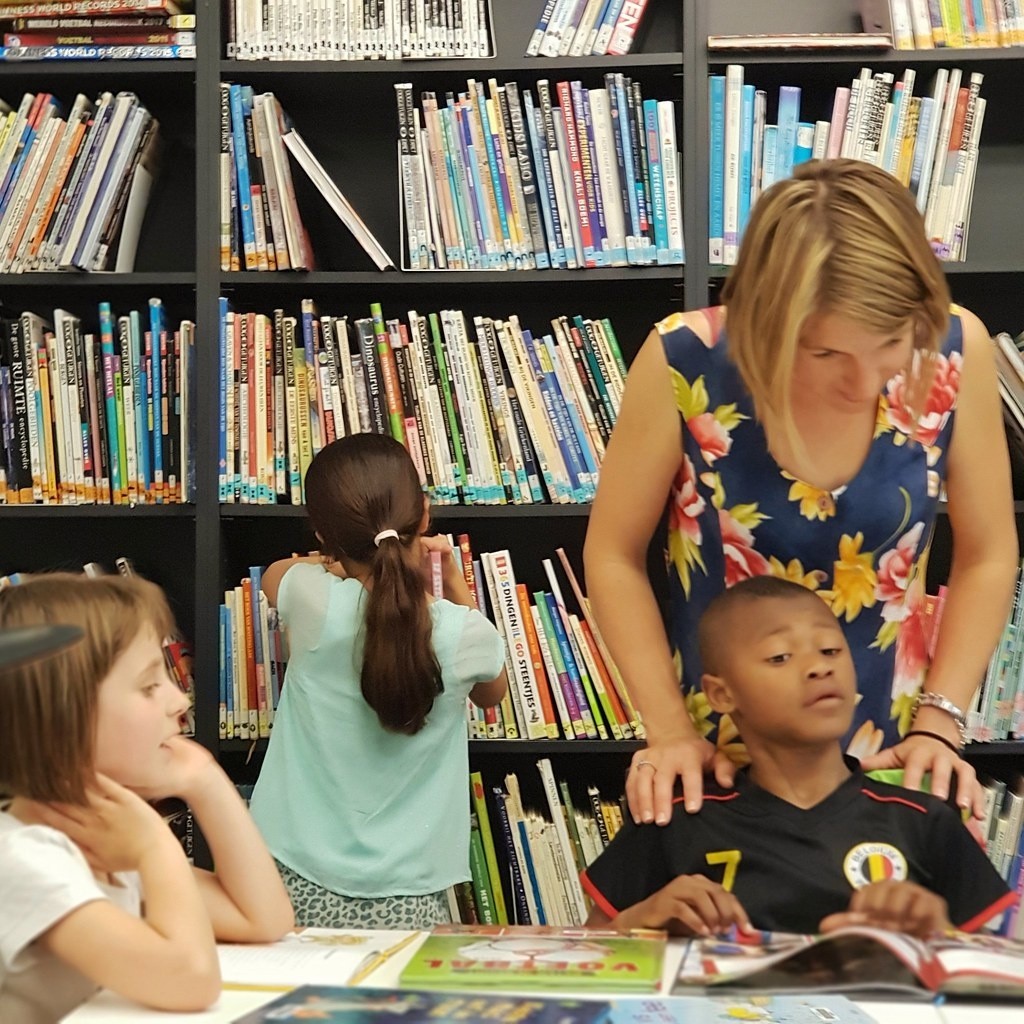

[991,330,1024,465]
[706,0,1024,52]
[220,0,686,926]
[0,0,199,507]
[708,63,987,266]
[84,556,197,864]
[920,583,1024,941]
[215,924,1024,1024]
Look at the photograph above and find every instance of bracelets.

[904,730,960,755]
[910,692,968,749]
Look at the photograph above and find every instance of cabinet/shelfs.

[0,1,1023,911]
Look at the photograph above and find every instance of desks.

[58,927,1024,1024]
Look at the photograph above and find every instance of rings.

[638,761,657,771]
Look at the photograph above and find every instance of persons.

[247,431,508,929]
[581,574,1017,937]
[582,159,1019,825]
[0,573,295,1024]
